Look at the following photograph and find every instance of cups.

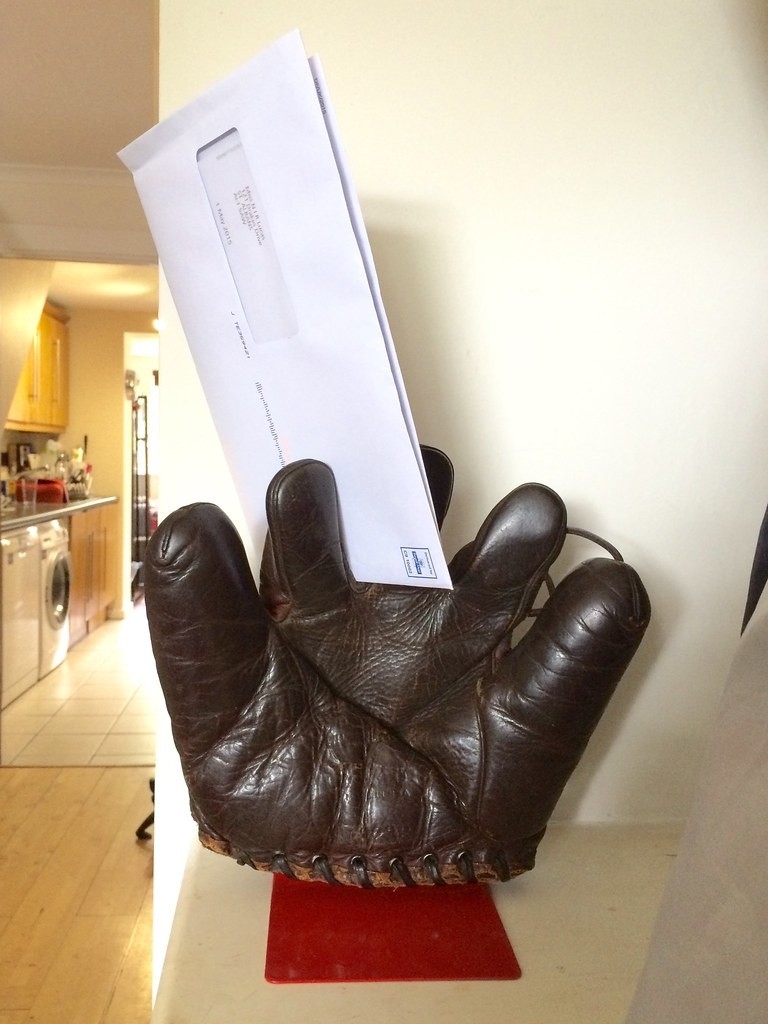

[22,479,37,506]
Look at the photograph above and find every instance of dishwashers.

[0,526,39,708]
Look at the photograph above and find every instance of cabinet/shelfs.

[3,311,70,434]
[68,506,117,652]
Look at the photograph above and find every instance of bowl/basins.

[27,454,58,470]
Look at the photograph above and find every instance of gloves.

[141,440,652,889]
